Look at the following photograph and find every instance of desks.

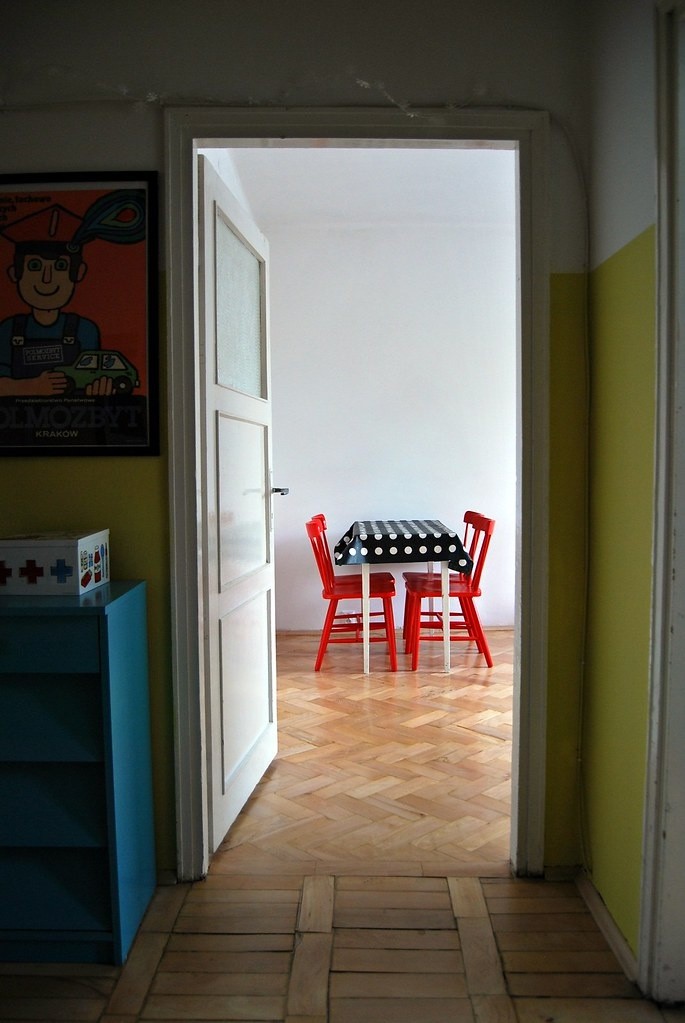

[333,520,473,673]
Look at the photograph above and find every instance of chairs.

[306,514,399,675]
[402,511,495,670]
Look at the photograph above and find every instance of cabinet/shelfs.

[0,580,155,966]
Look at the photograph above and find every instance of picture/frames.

[0,170,161,457]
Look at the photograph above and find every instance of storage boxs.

[0,528,110,596]
